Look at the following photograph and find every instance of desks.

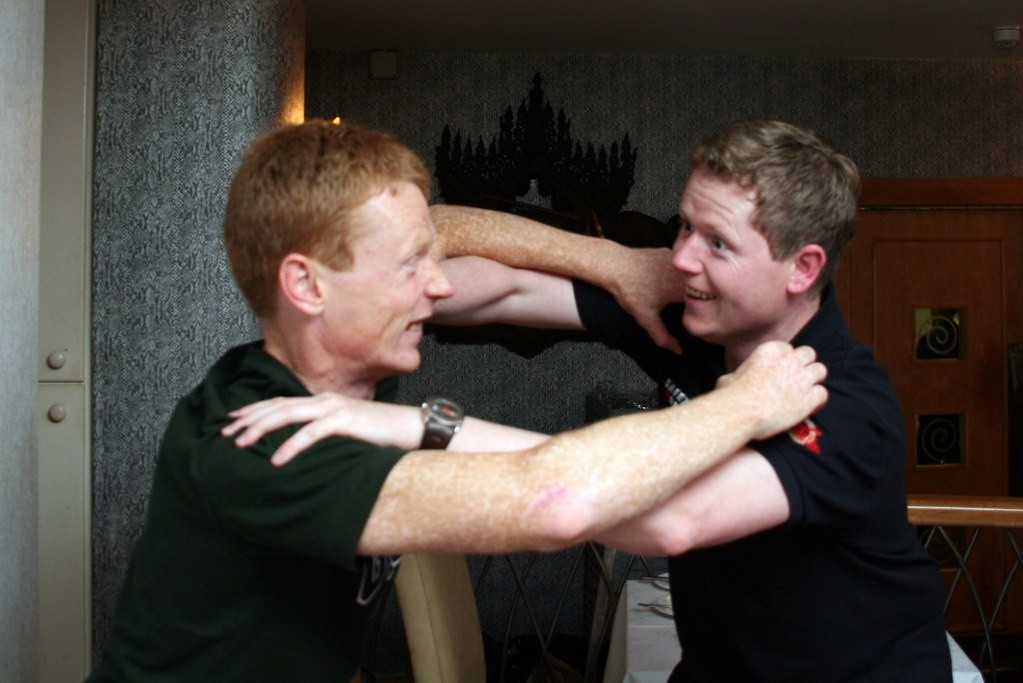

[906,493,1023,683]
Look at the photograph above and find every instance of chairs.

[395,550,486,683]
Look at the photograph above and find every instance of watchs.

[417,396,465,450]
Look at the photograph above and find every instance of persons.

[79,122,828,681]
[221,124,953,683]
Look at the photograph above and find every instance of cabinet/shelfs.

[835,178,1023,636]
